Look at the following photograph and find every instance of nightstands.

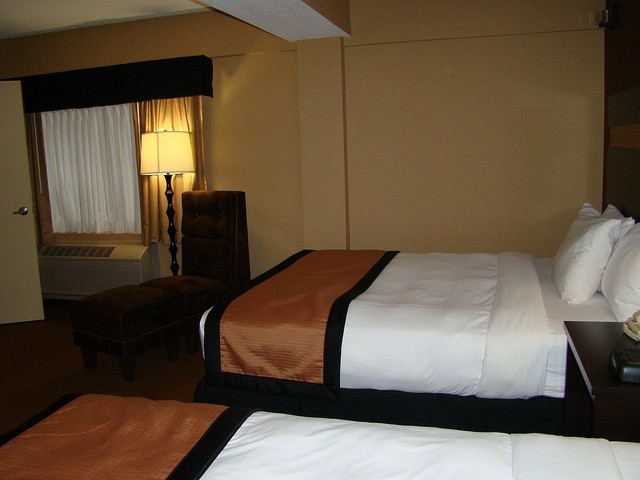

[563,321,640,441]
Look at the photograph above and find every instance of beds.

[197,247,622,402]
[0,392,640,479]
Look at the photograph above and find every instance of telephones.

[609,347,640,384]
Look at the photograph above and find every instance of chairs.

[68,190,251,382]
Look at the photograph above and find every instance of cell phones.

[611,343,639,385]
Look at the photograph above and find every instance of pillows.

[600,222,640,322]
[550,201,622,306]
[598,203,635,292]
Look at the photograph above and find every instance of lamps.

[139,129,198,277]
